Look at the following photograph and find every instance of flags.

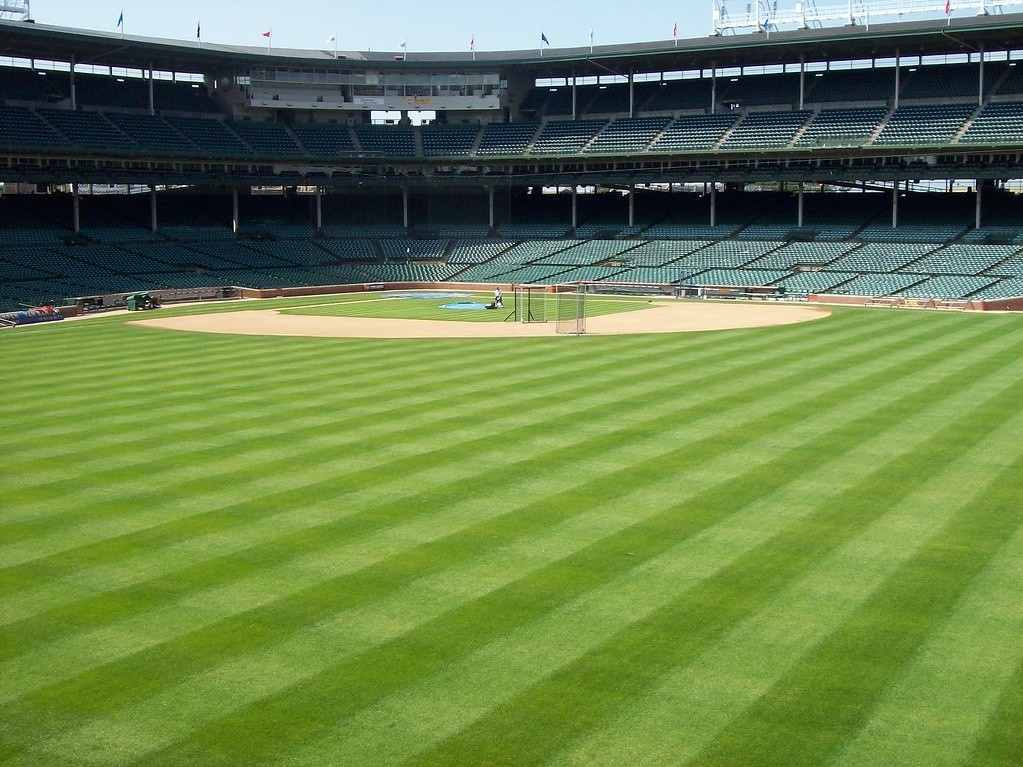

[763,19,770,31]
[542,33,550,45]
[673,22,677,37]
[196,23,201,38]
[400,39,408,49]
[326,35,335,43]
[261,31,271,37]
[470,38,473,50]
[945,0,950,15]
[589,29,593,42]
[116,12,123,27]
[864,11,869,25]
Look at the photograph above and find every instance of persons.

[495,287,503,305]
